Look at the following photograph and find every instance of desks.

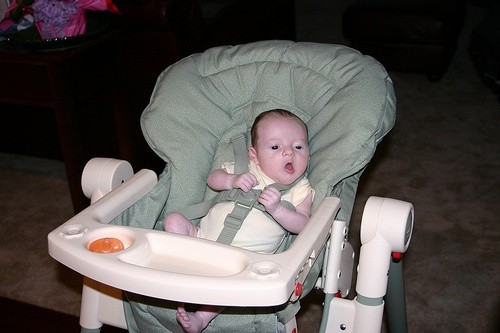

[0,14,136,218]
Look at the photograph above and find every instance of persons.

[162,109,315,333]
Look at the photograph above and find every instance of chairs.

[47,41,414,333]
